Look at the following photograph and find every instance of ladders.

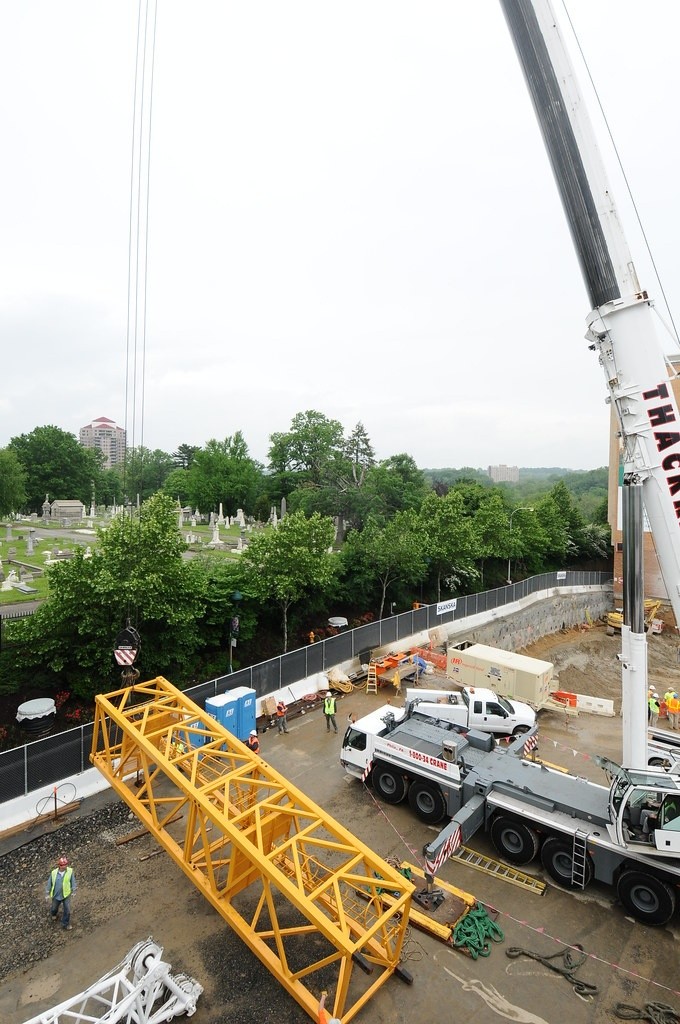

[450,845,549,897]
[366,661,377,695]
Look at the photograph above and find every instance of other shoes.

[335,730,338,733]
[65,924,72,930]
[284,731,289,733]
[327,729,330,732]
[52,916,56,920]
[279,731,283,735]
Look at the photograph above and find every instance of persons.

[246,730,259,775]
[650,693,660,727]
[45,858,77,930]
[648,685,656,720]
[666,694,680,730]
[323,692,338,733]
[640,794,676,838]
[277,699,289,735]
[664,687,676,715]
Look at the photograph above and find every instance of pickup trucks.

[406,687,537,742]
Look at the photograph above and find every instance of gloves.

[45,895,50,902]
[71,893,76,898]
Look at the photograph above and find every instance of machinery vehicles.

[606,599,662,638]
[114,0,680,926]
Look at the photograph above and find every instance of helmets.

[326,692,332,697]
[668,687,674,691]
[673,694,678,698]
[250,730,258,737]
[653,693,659,698]
[649,685,655,689]
[58,858,68,866]
[278,698,283,702]
